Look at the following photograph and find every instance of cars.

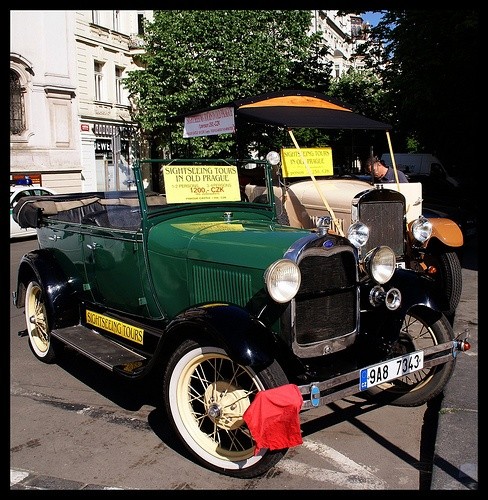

[10,179,58,240]
[14,155,471,479]
[236,97,464,317]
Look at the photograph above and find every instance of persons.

[361,154,410,186]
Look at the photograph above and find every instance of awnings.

[235,96,423,247]
